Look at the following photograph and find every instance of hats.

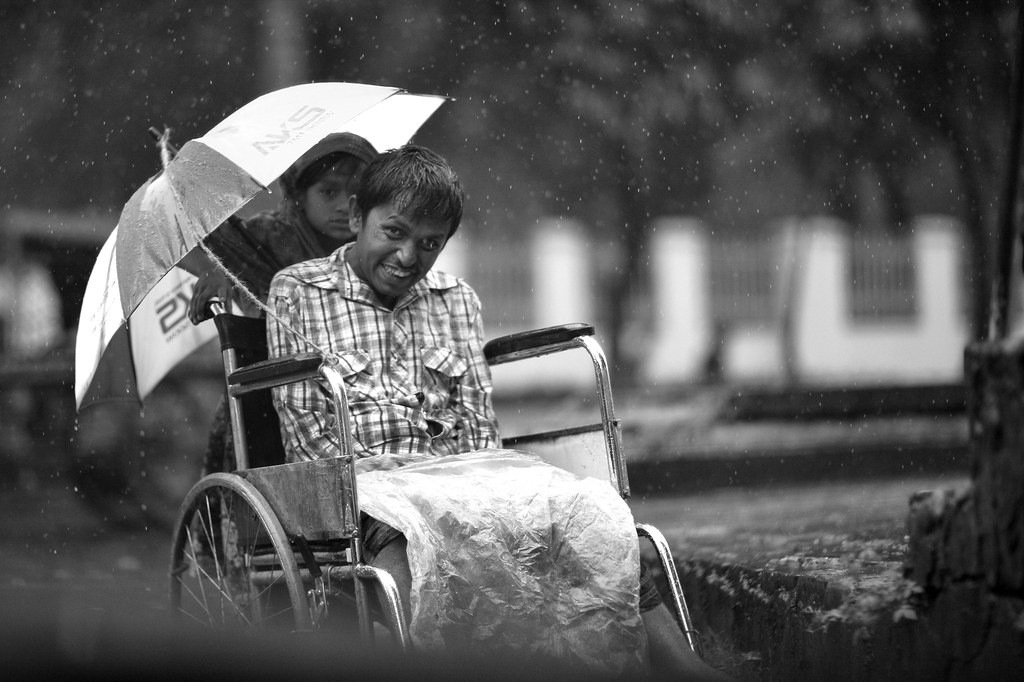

[278,132,379,199]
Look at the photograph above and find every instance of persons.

[265,144,728,682]
[177,132,376,579]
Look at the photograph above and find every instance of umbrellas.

[75,83,457,414]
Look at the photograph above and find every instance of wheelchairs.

[166,293,702,676]
